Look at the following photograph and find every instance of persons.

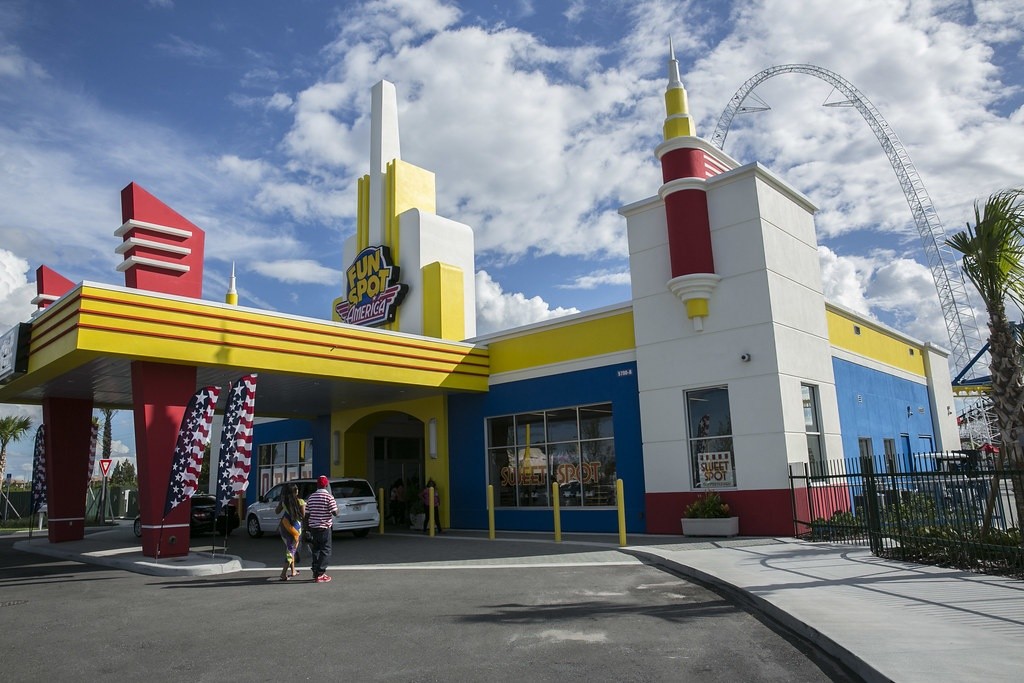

[305,476,338,583]
[275,483,306,580]
[419,479,446,535]
[386,477,418,526]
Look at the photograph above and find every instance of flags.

[698,414,709,453]
[86,424,99,493]
[213,371,257,522]
[31,424,47,516]
[163,385,222,517]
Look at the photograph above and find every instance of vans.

[246,477,381,541]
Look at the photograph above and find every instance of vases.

[681,516,739,536]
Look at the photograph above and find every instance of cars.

[133,493,240,538]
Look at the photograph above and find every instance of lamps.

[430,418,437,458]
[334,431,340,465]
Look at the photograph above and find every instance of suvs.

[917,476,984,511]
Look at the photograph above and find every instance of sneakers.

[315,574,332,583]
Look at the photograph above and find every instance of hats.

[317,474,329,489]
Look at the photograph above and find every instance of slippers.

[293,570,300,575]
[280,576,290,582]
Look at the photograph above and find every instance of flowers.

[683,490,731,518]
